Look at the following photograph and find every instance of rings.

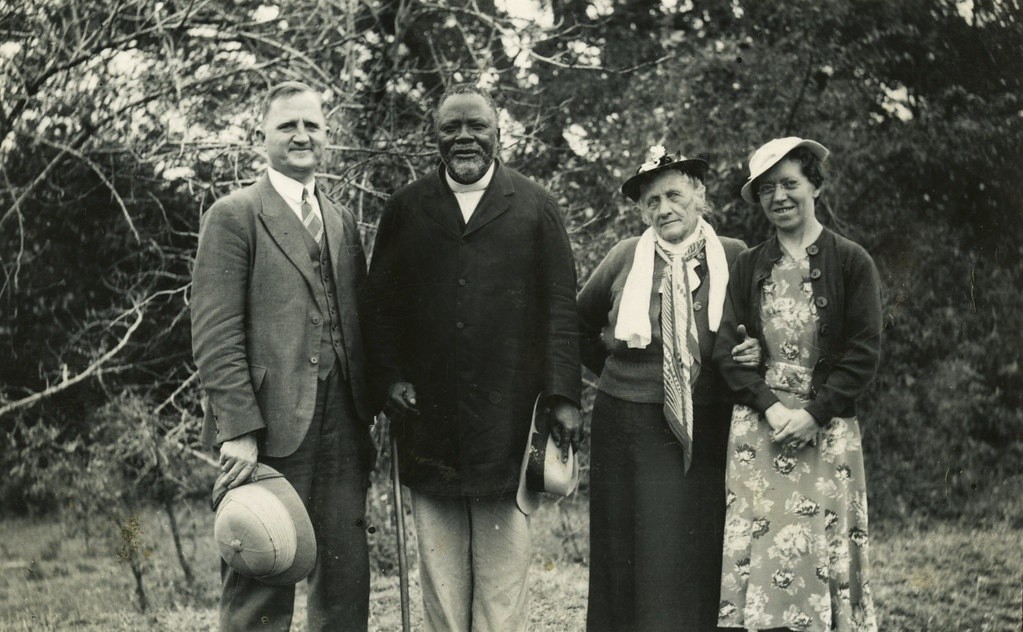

[790,441,798,448]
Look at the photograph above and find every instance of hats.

[622,154,709,202]
[211,462,318,585]
[515,392,579,515]
[740,136,829,205]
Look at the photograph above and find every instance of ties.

[302,188,323,243]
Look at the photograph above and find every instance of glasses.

[755,178,808,199]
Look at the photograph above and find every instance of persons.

[361,82,587,632]
[711,135,883,632]
[190,81,372,631]
[575,154,766,632]
